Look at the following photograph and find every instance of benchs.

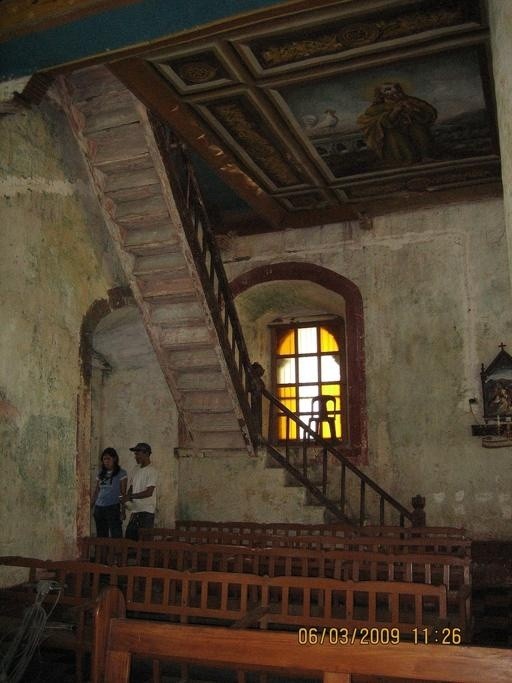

[0,521,511,682]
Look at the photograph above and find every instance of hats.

[129,443,152,454]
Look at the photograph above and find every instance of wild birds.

[310,109,339,137]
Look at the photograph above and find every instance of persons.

[91,447,128,538]
[120,442,157,540]
